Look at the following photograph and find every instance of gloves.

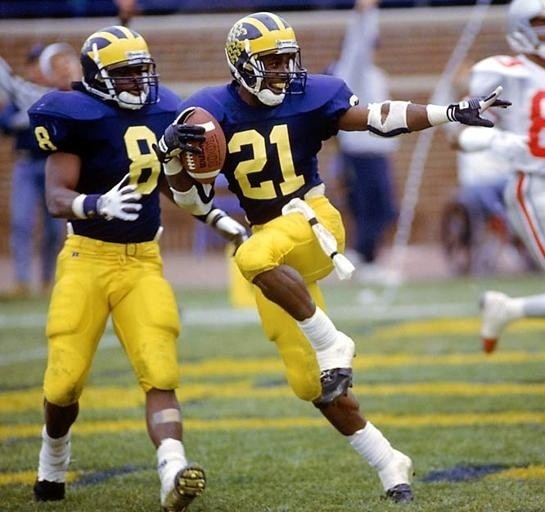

[455,87,510,127]
[491,130,529,163]
[206,208,248,257]
[154,107,206,160]
[85,174,144,225]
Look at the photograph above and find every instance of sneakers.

[315,333,356,403]
[479,291,510,354]
[381,449,414,505]
[161,460,204,512]
[34,447,68,502]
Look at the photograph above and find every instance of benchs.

[1,19,513,247]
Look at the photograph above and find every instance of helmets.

[506,1,545,59]
[81,26,159,110]
[224,12,307,106]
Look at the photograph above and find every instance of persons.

[469,0,545,352]
[28,26,248,512]
[152,12,512,503]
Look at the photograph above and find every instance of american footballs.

[180,108,228,185]
